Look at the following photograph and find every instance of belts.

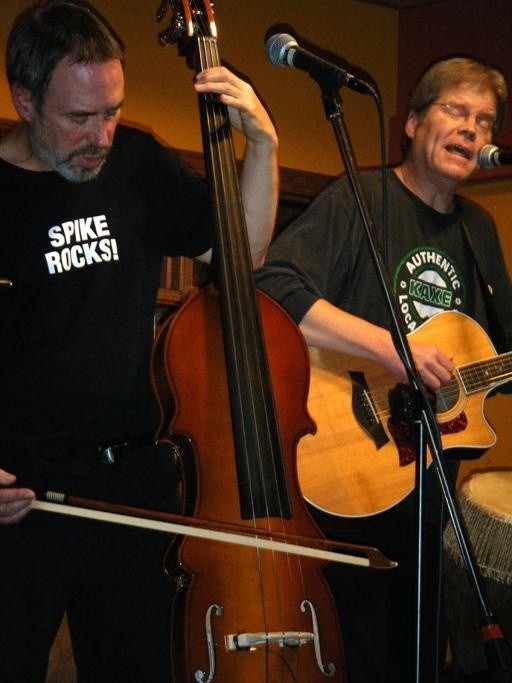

[44,433,161,463]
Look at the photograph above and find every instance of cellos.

[147,0,348,682]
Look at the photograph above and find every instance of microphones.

[267,33,374,119]
[478,143,511,171]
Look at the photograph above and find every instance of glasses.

[418,103,502,131]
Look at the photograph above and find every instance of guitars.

[298,309,511,517]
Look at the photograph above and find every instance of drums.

[443,463,512,585]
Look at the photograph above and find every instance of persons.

[0,0,281,678]
[250,54,512,678]
